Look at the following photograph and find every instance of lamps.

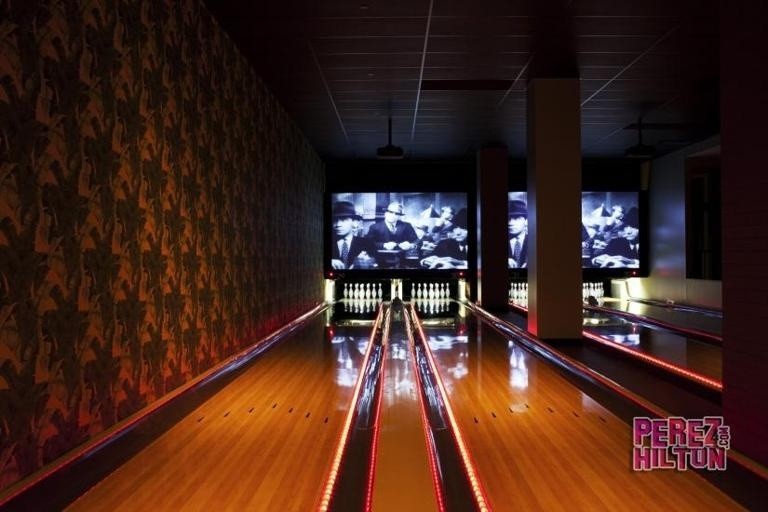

[375,117,406,160]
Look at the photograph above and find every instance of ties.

[632,243,638,259]
[461,244,466,261]
[390,224,395,234]
[342,239,348,268]
[513,238,521,267]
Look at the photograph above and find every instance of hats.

[332,201,362,220]
[619,207,639,229]
[382,201,405,216]
[509,200,527,219]
[449,206,468,228]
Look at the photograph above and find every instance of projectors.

[625,145,660,161]
[375,146,406,164]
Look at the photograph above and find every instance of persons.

[331,201,468,269]
[582,206,640,268]
[508,200,528,268]
